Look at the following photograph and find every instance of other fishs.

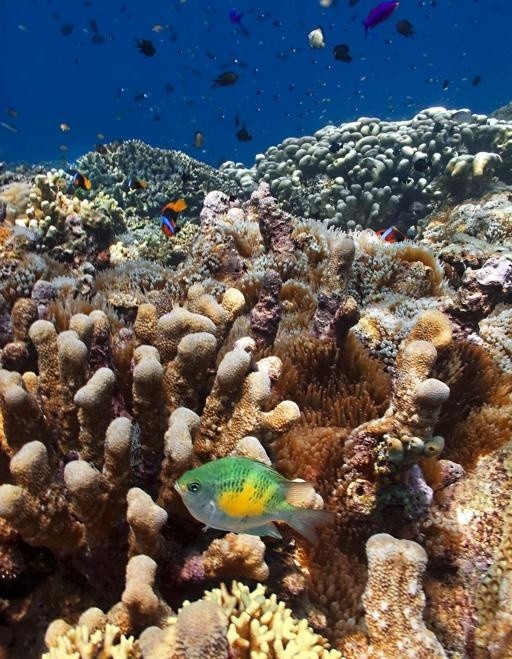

[52,0,481,155]
